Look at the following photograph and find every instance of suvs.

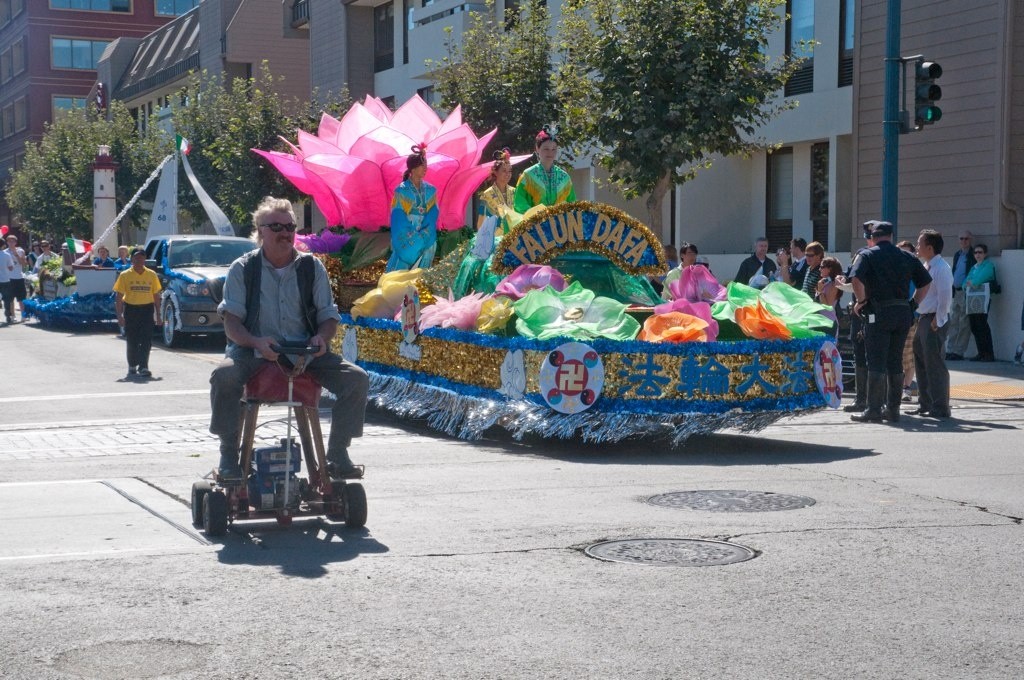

[451,236,670,312]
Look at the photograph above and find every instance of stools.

[242,362,331,496]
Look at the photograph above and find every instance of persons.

[889,238,919,405]
[835,219,880,413]
[908,227,956,418]
[0,238,14,325]
[511,122,577,214]
[3,236,31,323]
[56,242,68,257]
[849,220,935,424]
[1012,296,1024,366]
[91,246,114,268]
[35,240,60,269]
[384,152,440,272]
[111,246,164,377]
[661,210,864,339]
[939,229,977,362]
[206,193,372,486]
[962,242,1003,362]
[26,241,41,296]
[452,146,515,302]
[113,245,131,270]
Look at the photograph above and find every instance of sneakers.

[904,407,951,417]
[901,388,913,401]
[968,350,995,363]
[945,352,965,362]
[1013,344,1022,365]
[138,370,152,377]
[324,449,363,480]
[217,466,243,481]
[127,369,140,377]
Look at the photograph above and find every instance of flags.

[65,237,92,255]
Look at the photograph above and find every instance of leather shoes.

[843,397,866,412]
[850,406,883,424]
[881,407,901,422]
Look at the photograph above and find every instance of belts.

[878,298,910,307]
[920,313,936,318]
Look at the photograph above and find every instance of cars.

[141,234,261,347]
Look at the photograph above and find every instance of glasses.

[974,250,984,254]
[40,245,49,248]
[959,237,969,240]
[819,265,828,269]
[260,221,298,233]
[805,253,816,258]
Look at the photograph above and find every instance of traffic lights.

[914,60,943,126]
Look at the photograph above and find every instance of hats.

[871,221,895,239]
[862,219,880,239]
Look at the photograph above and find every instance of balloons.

[2,225,9,235]
